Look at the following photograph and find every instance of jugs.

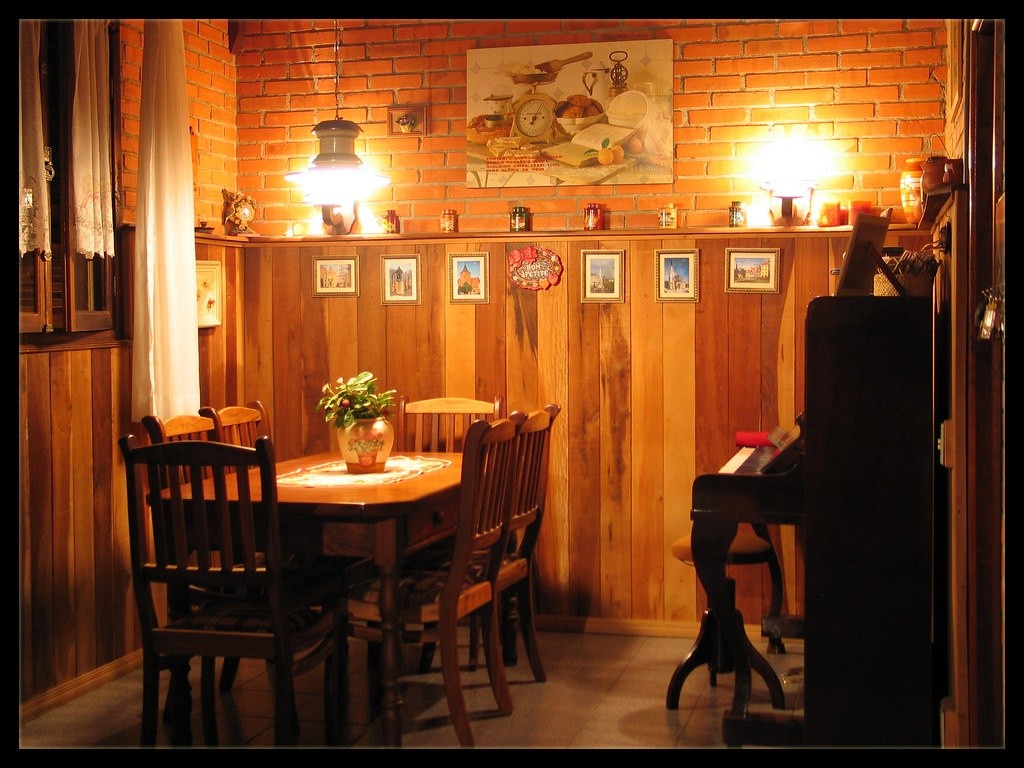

[582,60,612,108]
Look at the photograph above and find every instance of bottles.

[584,203,603,230]
[509,207,527,231]
[383,210,398,234]
[657,202,677,229]
[729,201,746,227]
[440,209,456,233]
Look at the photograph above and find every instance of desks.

[146,450,489,748]
[467,142,636,187]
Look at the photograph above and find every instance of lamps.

[284,18,393,206]
[762,183,817,226]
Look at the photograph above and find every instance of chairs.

[399,395,504,672]
[117,433,352,747]
[141,400,390,675]
[347,402,561,748]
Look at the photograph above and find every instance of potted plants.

[316,371,397,474]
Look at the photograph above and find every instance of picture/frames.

[196,260,222,329]
[833,213,909,295]
[387,105,427,138]
[581,249,625,303]
[653,248,701,303]
[724,247,780,293]
[311,254,360,298]
[448,251,490,305]
[379,253,422,306]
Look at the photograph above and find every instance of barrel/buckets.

[608,91,662,130]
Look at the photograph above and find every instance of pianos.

[685,292,940,749]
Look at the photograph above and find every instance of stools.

[665,531,786,714]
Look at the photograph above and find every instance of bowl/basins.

[555,110,606,134]
